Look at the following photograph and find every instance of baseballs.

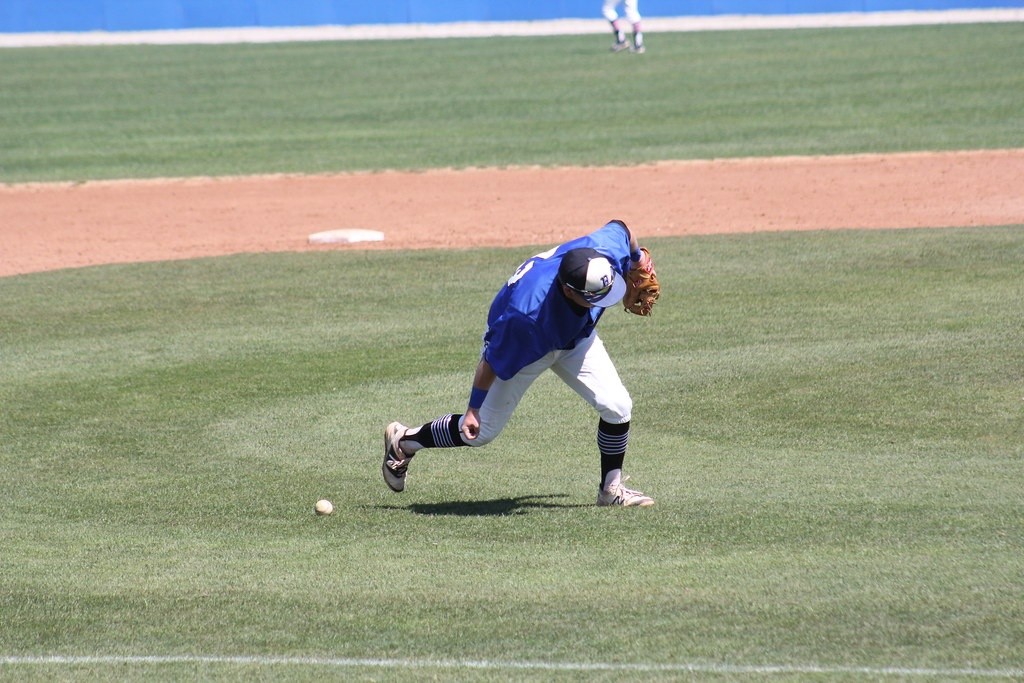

[315,499,333,516]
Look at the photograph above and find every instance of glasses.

[563,266,616,296]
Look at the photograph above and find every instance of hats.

[558,247,628,310]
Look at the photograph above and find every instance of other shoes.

[632,45,645,53]
[611,40,630,53]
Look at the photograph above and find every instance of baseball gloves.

[621,247,661,317]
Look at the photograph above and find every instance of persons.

[602,0,646,53]
[382,220,661,505]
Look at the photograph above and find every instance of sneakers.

[382,421,415,492]
[596,476,655,509]
